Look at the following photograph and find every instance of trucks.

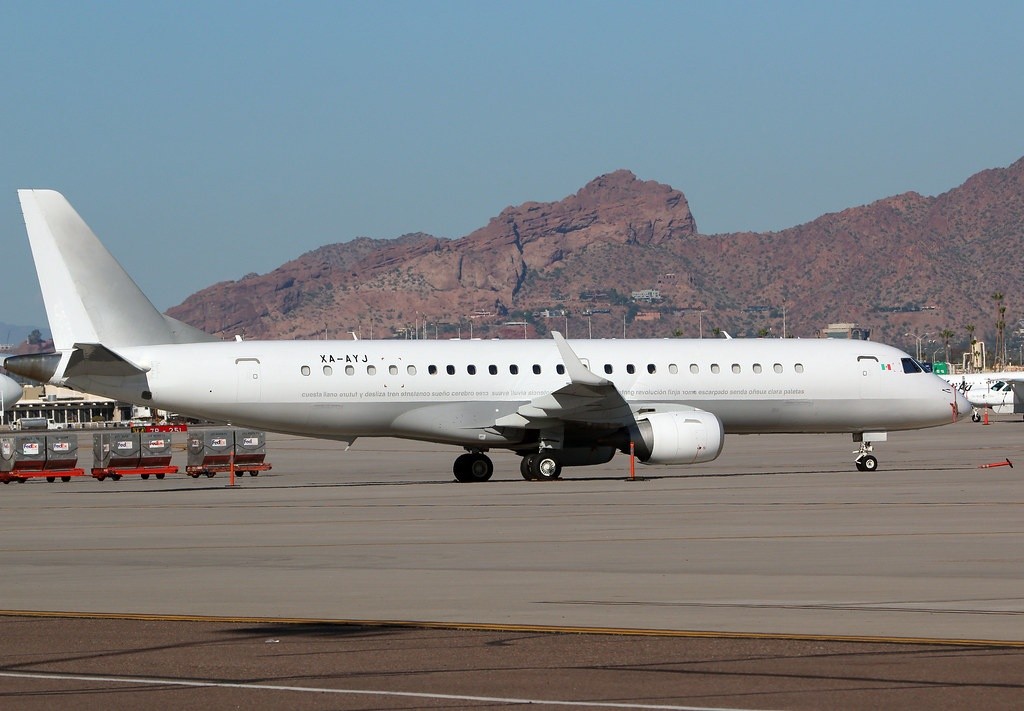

[16,417,64,431]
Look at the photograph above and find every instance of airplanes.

[3,188,974,483]
[938,372,1024,422]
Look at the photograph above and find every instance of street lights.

[934,348,944,364]
[905,332,929,362]
